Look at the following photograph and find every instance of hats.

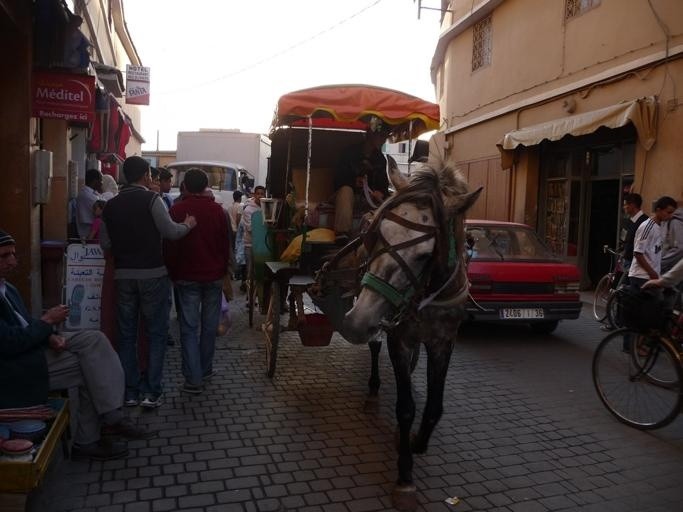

[0,231,16,247]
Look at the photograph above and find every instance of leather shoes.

[71,439,131,461]
[99,416,153,442]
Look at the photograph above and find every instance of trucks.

[162,128,273,233]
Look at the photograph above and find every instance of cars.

[458,219,584,343]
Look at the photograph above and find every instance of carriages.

[247,82,484,512]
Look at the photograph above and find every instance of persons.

[640,258,682,290]
[622,195,677,358]
[324,122,393,247]
[97,155,199,409]
[160,166,237,395]
[75,165,268,349]
[0,230,161,463]
[650,196,682,276]
[604,192,649,331]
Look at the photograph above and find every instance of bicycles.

[590,243,682,429]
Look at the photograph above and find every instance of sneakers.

[181,384,203,394]
[203,369,218,381]
[127,399,138,407]
[139,395,165,409]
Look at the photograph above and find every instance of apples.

[216,325,225,336]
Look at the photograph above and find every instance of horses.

[341,153,484,512]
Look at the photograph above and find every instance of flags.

[123,64,150,106]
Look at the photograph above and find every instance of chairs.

[292,164,365,233]
